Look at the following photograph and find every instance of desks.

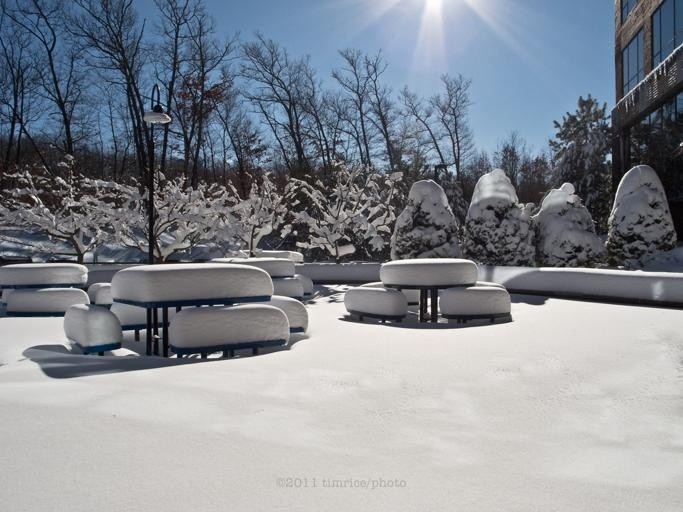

[384,284,475,323]
[0,284,84,289]
[271,276,293,278]
[113,296,271,357]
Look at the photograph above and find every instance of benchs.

[75,323,303,360]
[304,293,312,296]
[91,301,111,309]
[6,311,65,316]
[293,296,303,300]
[350,302,509,327]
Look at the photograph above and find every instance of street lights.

[144,83,171,264]
[435,163,447,182]
